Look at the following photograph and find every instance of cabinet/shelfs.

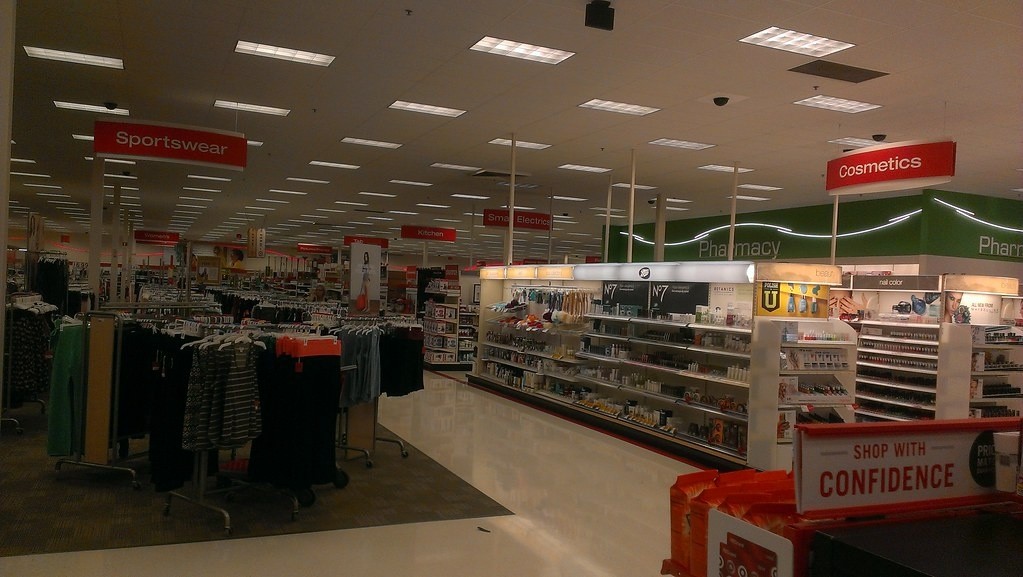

[465,262,858,472]
[829,274,1023,423]
[420,276,480,371]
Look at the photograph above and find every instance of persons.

[360,252,371,313]
[829,290,868,315]
[192,254,198,271]
[312,285,329,302]
[944,292,963,323]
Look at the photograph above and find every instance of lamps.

[584,0,615,32]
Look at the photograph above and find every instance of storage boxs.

[424,278,473,363]
[971,351,985,372]
[779,376,798,405]
[972,326,985,345]
[777,409,796,443]
[970,377,984,398]
[969,408,983,419]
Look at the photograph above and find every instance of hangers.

[7,286,423,351]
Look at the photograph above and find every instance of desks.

[808,511,1023,576]
